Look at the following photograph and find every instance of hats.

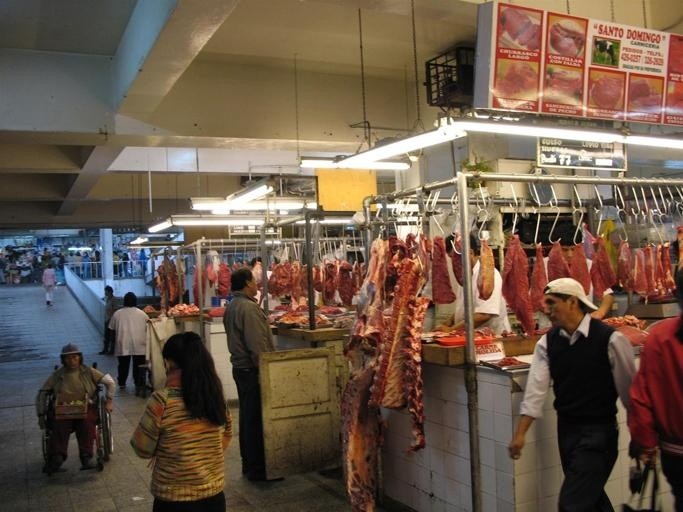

[543,277,600,313]
[60,342,82,356]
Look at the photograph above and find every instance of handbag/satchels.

[623,462,663,512]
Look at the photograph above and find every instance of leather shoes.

[242,463,287,483]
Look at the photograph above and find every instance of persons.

[130,330,232,507]
[625,264,683,512]
[506,277,635,512]
[34,343,114,474]
[224,269,286,481]
[98,285,119,357]
[43,264,57,306]
[109,292,151,398]
[0,244,153,286]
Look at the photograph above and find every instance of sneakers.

[47,456,63,470]
[81,458,97,467]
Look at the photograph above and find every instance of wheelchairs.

[42,362,113,472]
